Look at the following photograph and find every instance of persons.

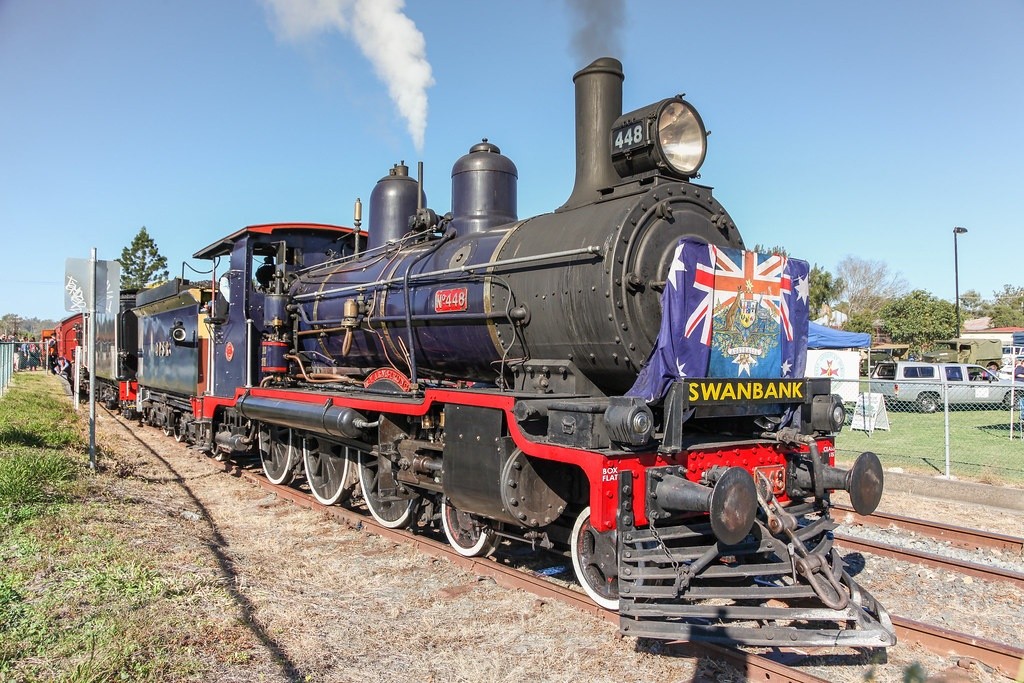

[1,331,71,377]
[983,365,998,380]
[1014,359,1024,382]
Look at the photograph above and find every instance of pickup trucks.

[870,360,1024,414]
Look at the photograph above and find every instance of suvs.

[860,343,909,376]
[921,338,1005,373]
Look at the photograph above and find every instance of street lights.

[953,226,969,338]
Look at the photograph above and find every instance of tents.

[806,320,873,439]
[1009,331,1024,443]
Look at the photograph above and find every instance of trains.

[40,56,899,648]
